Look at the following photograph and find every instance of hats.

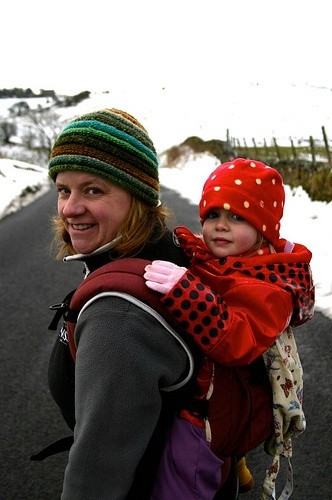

[48,108,159,207]
[199,158,285,246]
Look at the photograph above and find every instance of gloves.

[143,260,188,295]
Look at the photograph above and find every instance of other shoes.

[236,457,254,494]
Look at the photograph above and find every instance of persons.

[143,158,315,500]
[46,107,241,500]
